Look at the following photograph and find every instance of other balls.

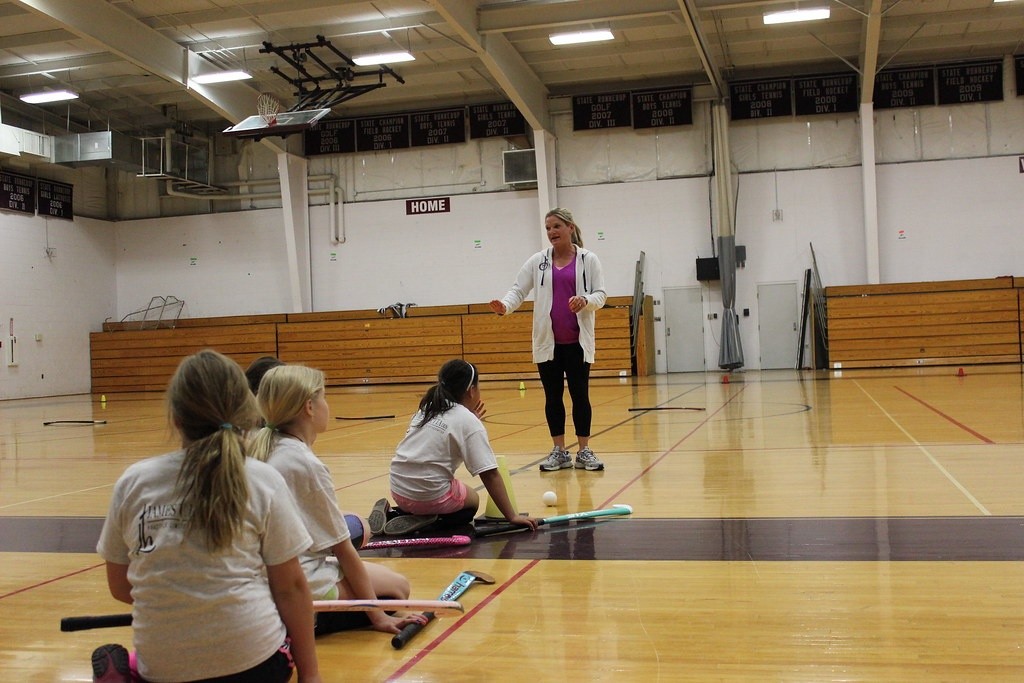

[543,491,557,506]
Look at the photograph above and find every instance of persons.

[246,355,370,547]
[489,207,606,471]
[368,359,538,532]
[249,365,427,634]
[541,471,619,682]
[90,350,322,683]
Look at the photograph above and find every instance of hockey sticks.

[474,504,634,536]
[628,407,705,412]
[43,420,107,426]
[334,415,396,421]
[390,570,495,648]
[58,599,467,633]
[365,534,472,549]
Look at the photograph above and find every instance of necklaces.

[277,431,303,442]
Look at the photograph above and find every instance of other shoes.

[279,637,295,668]
[91,644,138,683]
[384,512,439,536]
[368,498,389,534]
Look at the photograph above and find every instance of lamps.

[549,22,615,46]
[19,69,80,104]
[761,0,831,25]
[352,29,416,66]
[192,47,255,84]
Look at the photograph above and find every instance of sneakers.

[574,448,604,471]
[539,446,573,471]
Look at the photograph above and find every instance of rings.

[579,304,581,307]
